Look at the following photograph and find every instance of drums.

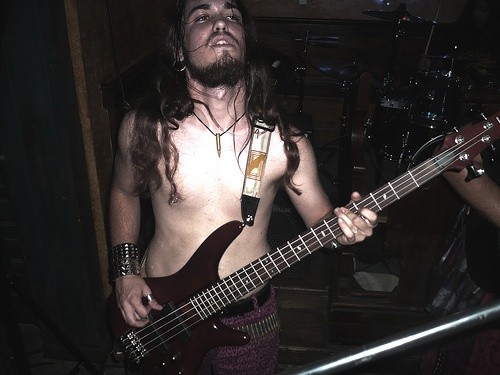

[365,103,497,226]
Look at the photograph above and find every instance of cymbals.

[423,46,481,63]
[361,3,437,26]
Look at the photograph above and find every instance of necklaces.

[348,235,355,242]
[193,110,246,158]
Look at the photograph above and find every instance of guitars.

[104,111,500,375]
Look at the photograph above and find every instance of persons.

[105,0,378,375]
[412,118,500,375]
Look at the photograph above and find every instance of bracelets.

[112,243,142,278]
